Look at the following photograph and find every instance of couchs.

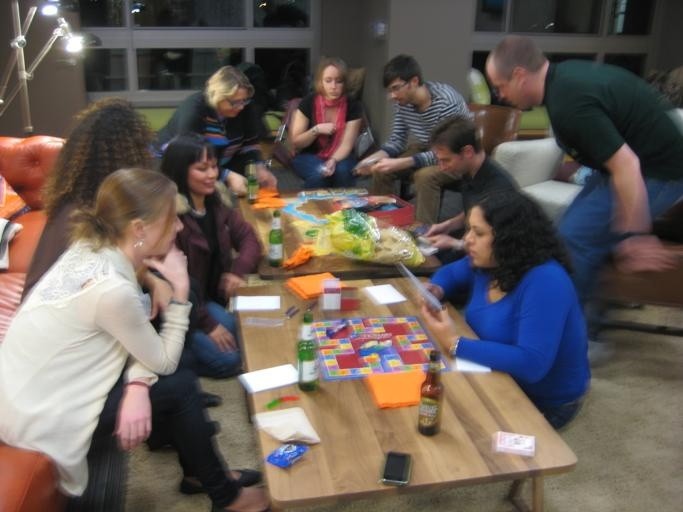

[137,109,282,134]
[0,136,72,512]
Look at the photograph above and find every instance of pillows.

[0,217,24,270]
[0,174,31,220]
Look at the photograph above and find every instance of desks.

[234,190,443,278]
[233,276,579,512]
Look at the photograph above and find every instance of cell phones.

[382,451,412,486]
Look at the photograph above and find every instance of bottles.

[296,310,318,392]
[268,210,284,267]
[247,160,258,204]
[417,350,444,436]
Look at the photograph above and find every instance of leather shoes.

[181,469,271,512]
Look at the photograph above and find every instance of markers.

[329,323,347,336]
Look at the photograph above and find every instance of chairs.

[401,104,522,222]
[467,71,551,136]
[495,106,682,229]
[586,194,683,341]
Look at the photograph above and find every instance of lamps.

[0,0,83,132]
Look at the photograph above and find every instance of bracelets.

[124,381,149,388]
[449,337,459,355]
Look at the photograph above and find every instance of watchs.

[309,126,320,136]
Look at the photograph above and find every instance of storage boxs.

[364,192,416,227]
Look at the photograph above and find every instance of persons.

[21,98,157,299]
[357,55,469,224]
[485,35,682,342]
[420,115,520,263]
[153,65,277,197]
[1,167,271,510]
[289,57,363,187]
[419,188,591,430]
[158,132,263,379]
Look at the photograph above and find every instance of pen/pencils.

[285,304,300,320]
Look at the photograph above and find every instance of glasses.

[227,98,251,107]
